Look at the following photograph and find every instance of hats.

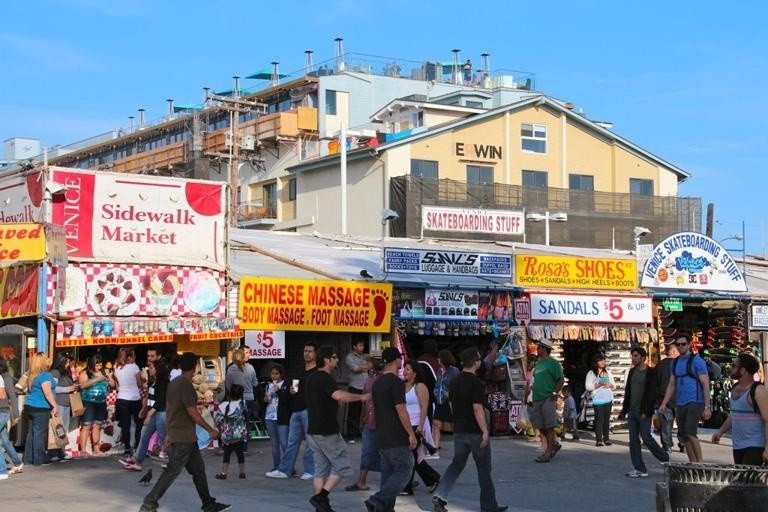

[405,321,481,336]
[382,347,403,363]
[536,339,554,350]
[426,307,476,315]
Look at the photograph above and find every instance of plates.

[90,270,141,317]
[184,273,219,314]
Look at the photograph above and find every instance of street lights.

[718,232,743,247]
[238,202,263,213]
[523,209,570,246]
[337,120,377,237]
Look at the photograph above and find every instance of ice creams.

[93,272,136,315]
[273,377,277,385]
[142,268,180,315]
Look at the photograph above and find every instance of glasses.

[674,342,686,346]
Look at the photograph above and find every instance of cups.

[292,379,299,392]
[266,392,272,401]
[151,290,177,314]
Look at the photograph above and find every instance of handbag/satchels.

[47,413,68,450]
[479,292,526,411]
[68,391,86,416]
[400,292,425,319]
[196,406,219,450]
[427,291,437,306]
[15,370,29,390]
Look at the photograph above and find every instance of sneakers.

[0,452,107,480]
[301,473,313,480]
[266,469,288,479]
[528,436,687,478]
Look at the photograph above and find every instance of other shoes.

[492,506,507,512]
[310,494,333,512]
[364,500,375,512]
[120,457,135,466]
[430,481,439,493]
[216,472,227,480]
[240,473,245,479]
[206,502,233,512]
[125,464,141,471]
[432,496,448,512]
[399,491,409,495]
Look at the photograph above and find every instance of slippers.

[345,483,369,491]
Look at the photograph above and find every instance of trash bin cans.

[662,460,768,512]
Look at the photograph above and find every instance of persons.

[299,343,373,512]
[1,344,172,479]
[136,349,233,512]
[430,345,508,511]
[522,339,712,478]
[362,347,424,512]
[710,342,768,468]
[210,345,319,481]
[344,340,502,494]
